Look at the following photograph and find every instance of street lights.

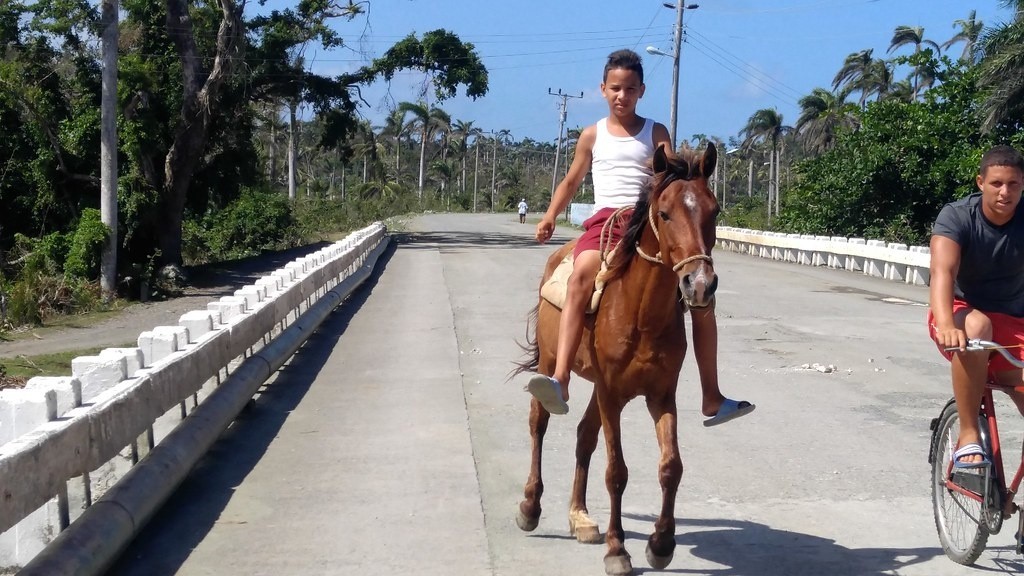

[647,45,679,155]
[548,86,584,204]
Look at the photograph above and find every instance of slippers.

[528,374,569,414]
[953,444,991,469]
[704,399,756,426]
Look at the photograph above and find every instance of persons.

[928,146,1024,469]
[528,50,751,417]
[518,199,528,224]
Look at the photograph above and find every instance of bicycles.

[928,336,1024,567]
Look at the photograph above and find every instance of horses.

[507,142,722,575]
